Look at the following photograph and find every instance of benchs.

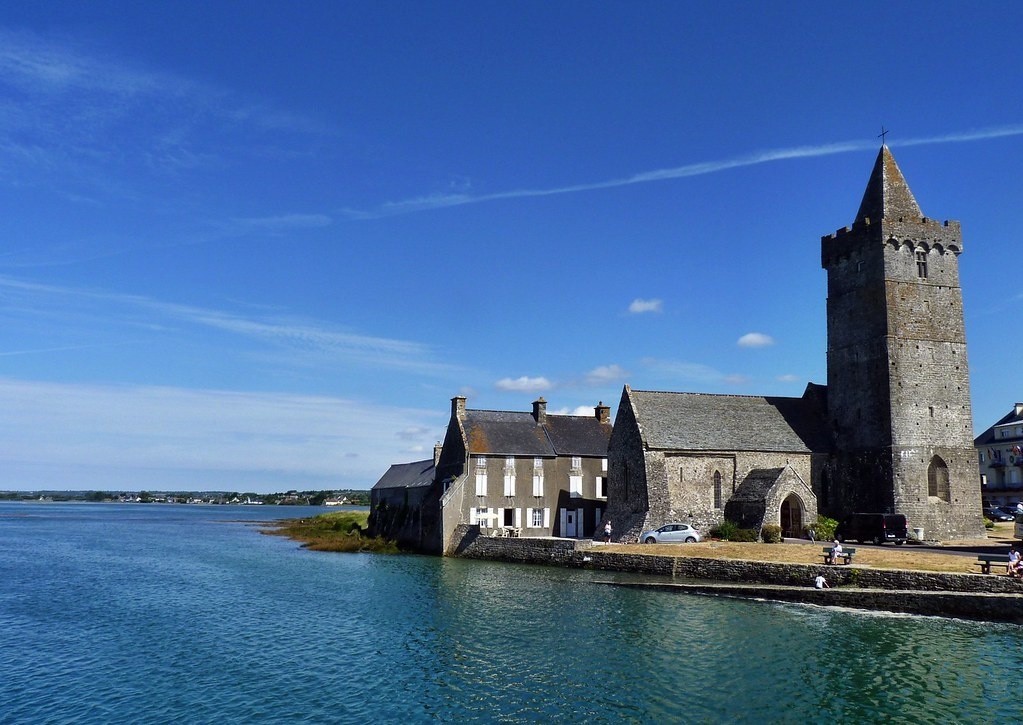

[818,547,856,565]
[973,554,1011,575]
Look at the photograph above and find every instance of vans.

[834,513,908,545]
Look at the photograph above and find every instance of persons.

[814,571,829,589]
[829,540,842,565]
[604,521,612,546]
[1007,547,1023,578]
[809,528,814,544]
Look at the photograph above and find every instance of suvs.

[983,500,1002,510]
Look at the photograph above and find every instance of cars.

[1004,502,1023,510]
[983,508,1014,522]
[641,522,701,544]
[1000,506,1023,521]
